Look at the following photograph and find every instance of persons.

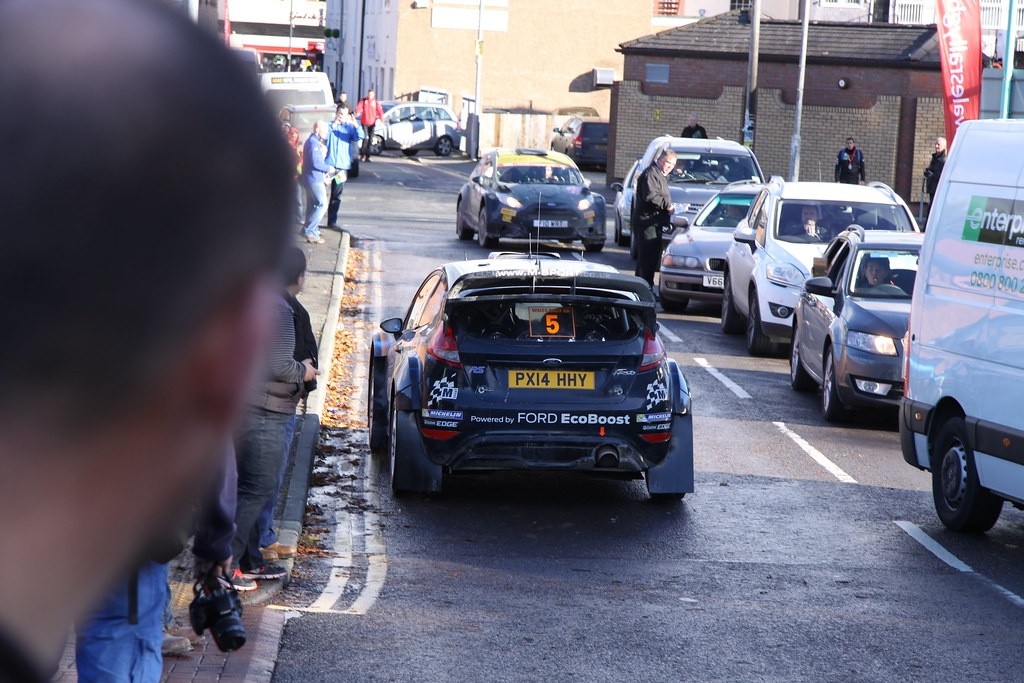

[356,88,385,162]
[234,297,319,591]
[632,150,676,300]
[279,105,303,224]
[836,137,865,184]
[74,425,237,683]
[682,115,708,168]
[857,254,893,287]
[335,91,351,114]
[256,243,318,560]
[670,159,692,180]
[0,0,297,683]
[924,137,948,232]
[788,205,830,241]
[325,105,364,228]
[303,120,336,243]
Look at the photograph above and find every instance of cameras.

[191,589,247,652]
[924,168,931,177]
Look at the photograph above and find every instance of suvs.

[724,175,922,355]
[628,132,766,260]
[551,114,608,171]
[656,177,766,313]
[281,103,359,177]
[788,224,925,422]
[358,101,462,156]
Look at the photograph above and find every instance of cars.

[456,147,607,252]
[611,159,641,246]
[369,250,695,516]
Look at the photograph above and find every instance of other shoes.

[650,293,660,302]
[359,159,372,162]
[298,220,305,225]
[328,224,341,229]
[300,227,325,245]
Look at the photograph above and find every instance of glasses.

[662,159,676,166]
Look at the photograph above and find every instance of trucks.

[257,71,334,115]
[241,42,325,71]
[898,116,1024,539]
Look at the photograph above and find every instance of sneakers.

[258,541,296,562]
[218,568,257,590]
[243,563,287,578]
[161,624,205,653]
